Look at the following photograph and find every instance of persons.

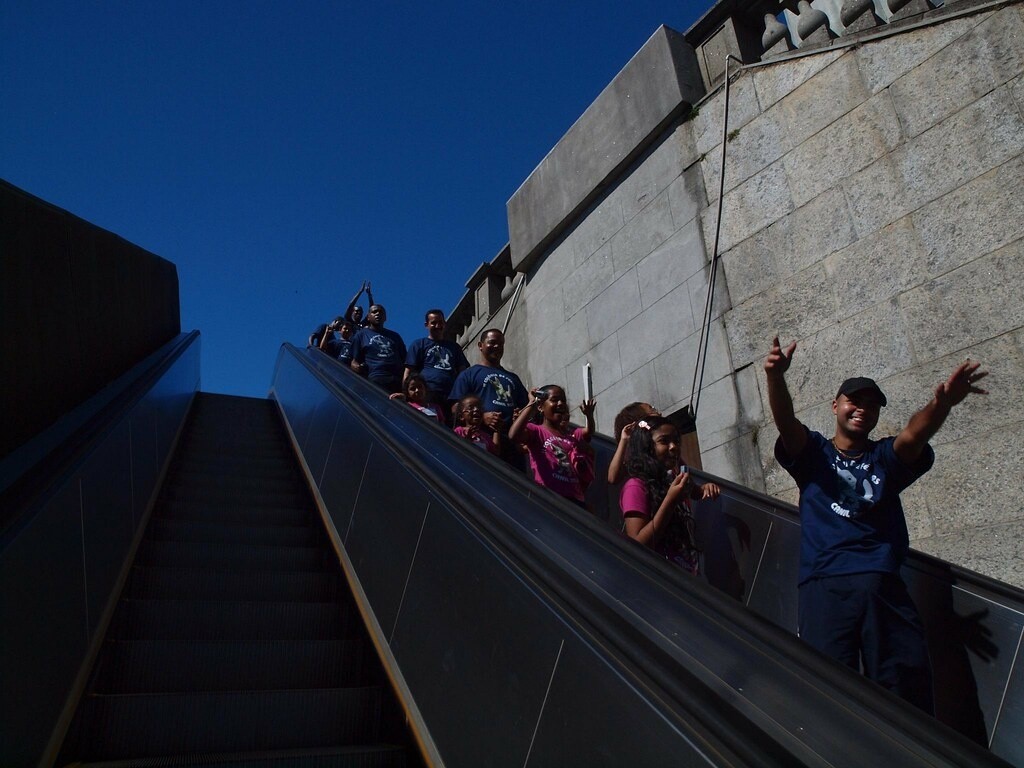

[764,337,989,720]
[351,304,408,395]
[389,375,445,426]
[618,416,720,583]
[447,329,529,468]
[308,316,343,349]
[402,309,470,420]
[344,280,374,340]
[608,402,687,485]
[508,385,593,514]
[512,399,597,457]
[451,395,502,456]
[325,323,352,359]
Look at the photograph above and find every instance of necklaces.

[832,437,869,459]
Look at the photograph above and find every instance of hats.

[836,377,887,406]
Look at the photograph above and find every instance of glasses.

[644,406,657,415]
[461,404,482,415]
